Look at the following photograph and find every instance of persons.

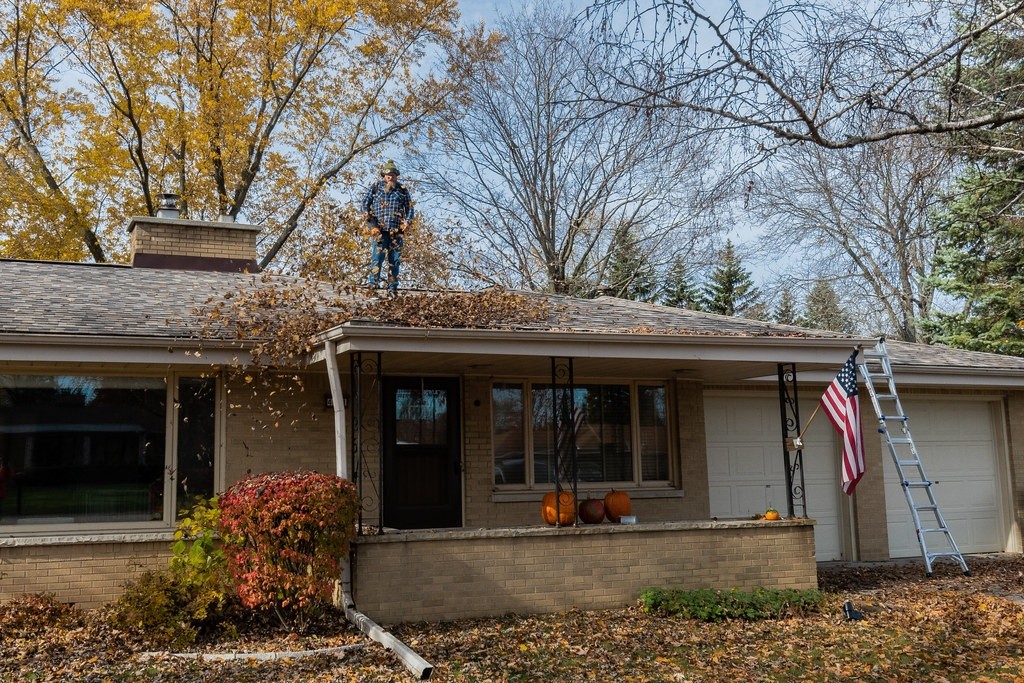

[360,159,414,290]
[0,455,15,516]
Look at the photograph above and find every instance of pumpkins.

[541,488,576,525]
[765,508,779,520]
[604,488,632,523]
[578,497,604,523]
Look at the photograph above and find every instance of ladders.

[857,334,971,577]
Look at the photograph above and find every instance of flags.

[552,397,584,480]
[820,349,865,497]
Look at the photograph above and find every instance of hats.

[380,159,400,177]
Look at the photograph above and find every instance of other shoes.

[369,291,382,301]
[386,292,399,300]
